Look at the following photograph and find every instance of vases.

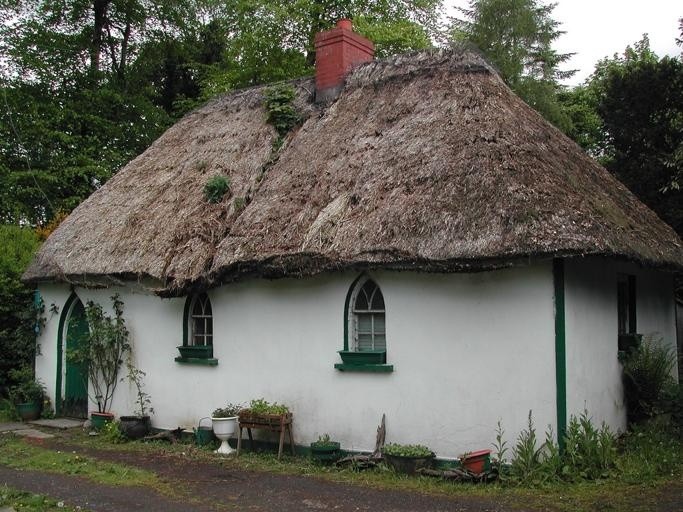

[457,446,492,475]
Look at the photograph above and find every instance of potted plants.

[65,292,132,429]
[238,401,291,430]
[310,435,342,465]
[116,351,153,439]
[15,380,46,421]
[382,440,436,474]
[210,402,240,455]
